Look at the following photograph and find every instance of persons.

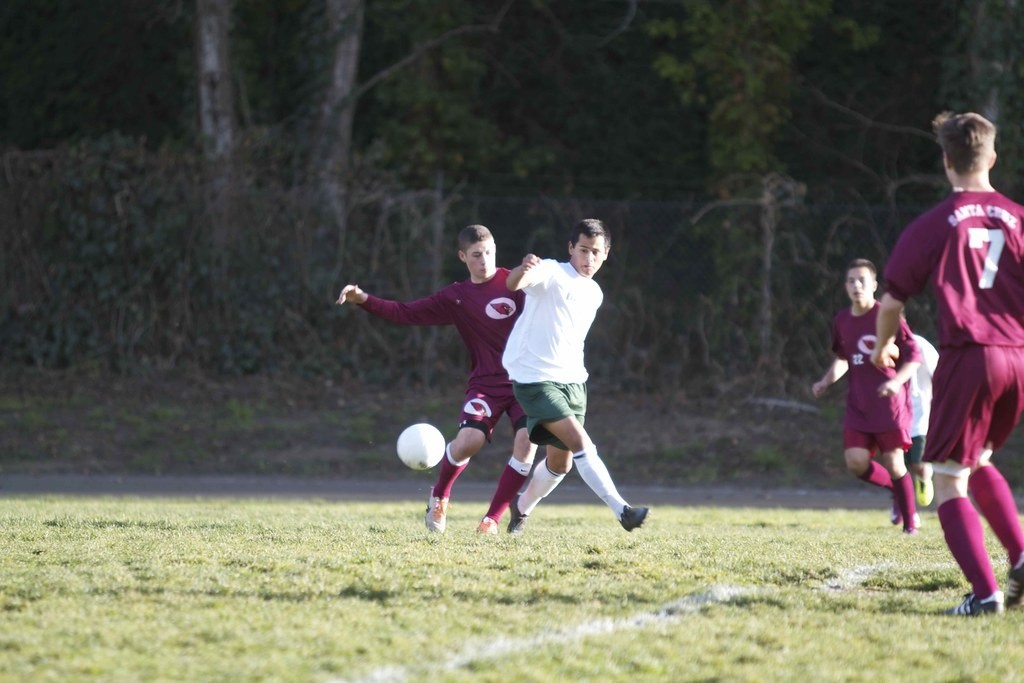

[501,219,651,538]
[811,257,923,535]
[870,109,1023,617]
[335,224,540,538]
[900,306,939,509]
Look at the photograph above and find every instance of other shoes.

[890,494,902,525]
[902,528,918,536]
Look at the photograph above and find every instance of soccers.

[396,421,448,472]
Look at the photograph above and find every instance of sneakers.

[476,516,498,536]
[507,492,529,535]
[426,486,449,533]
[915,462,935,508]
[1004,558,1024,608]
[619,505,650,532]
[947,591,1005,616]
